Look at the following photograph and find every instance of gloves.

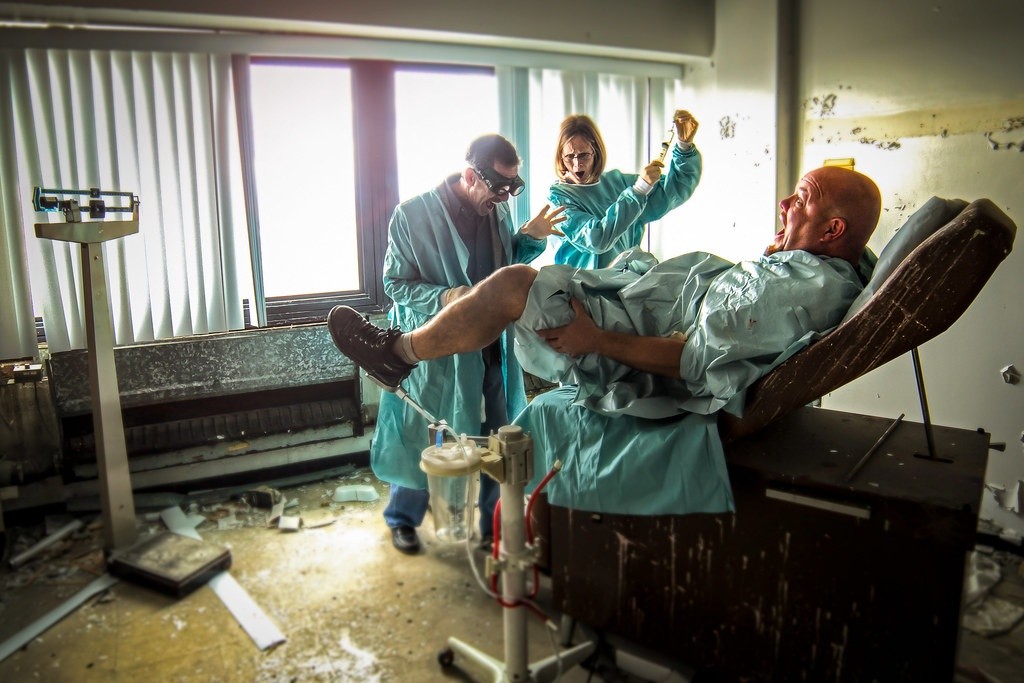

[446,286,473,305]
[640,161,664,186]
[673,110,699,142]
[521,204,567,240]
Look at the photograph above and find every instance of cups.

[419,442,483,544]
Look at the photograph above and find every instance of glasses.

[472,164,525,197]
[561,151,594,160]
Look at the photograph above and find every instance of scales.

[32,186,233,600]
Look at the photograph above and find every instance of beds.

[715,194,1017,464]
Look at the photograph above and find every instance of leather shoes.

[392,526,420,554]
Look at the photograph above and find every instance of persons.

[366,132,569,555]
[324,167,882,394]
[543,108,703,271]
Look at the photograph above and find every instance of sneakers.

[327,305,419,387]
